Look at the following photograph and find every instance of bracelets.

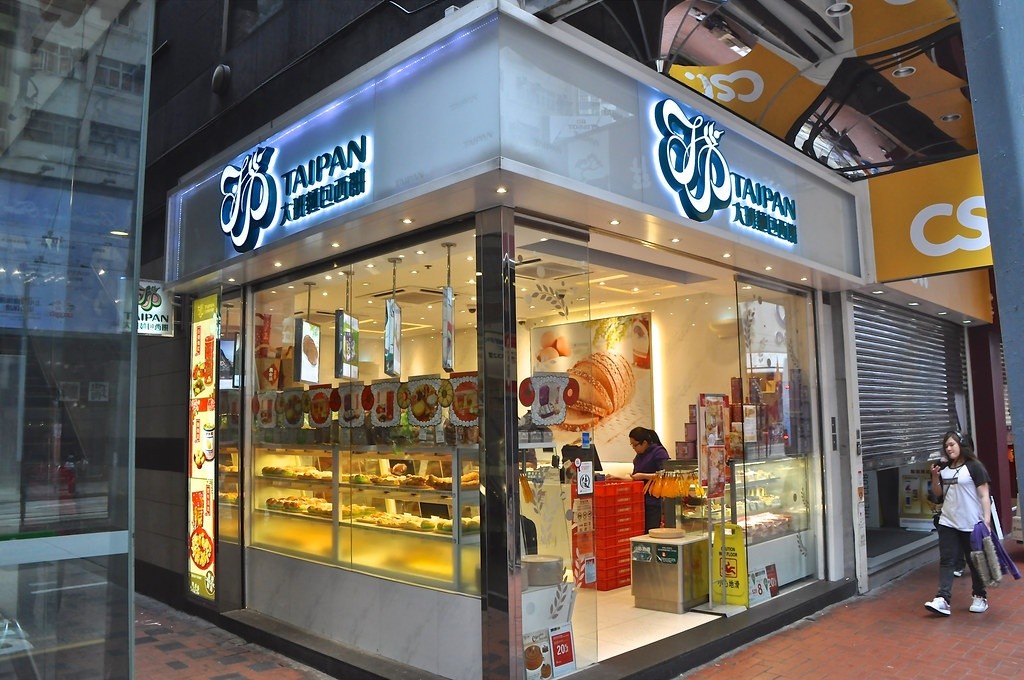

[932,481,941,486]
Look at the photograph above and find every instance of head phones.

[954,430,968,447]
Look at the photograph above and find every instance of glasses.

[630,441,643,448]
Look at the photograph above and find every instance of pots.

[474,553,567,592]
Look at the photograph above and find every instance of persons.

[924,430,991,616]
[628,427,672,535]
[945,434,976,577]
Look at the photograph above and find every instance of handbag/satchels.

[932,511,942,529]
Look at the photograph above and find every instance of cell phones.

[934,458,950,469]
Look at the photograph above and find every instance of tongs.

[641,469,704,498]
[519,472,536,504]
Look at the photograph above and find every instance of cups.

[202,425,215,461]
[204,335,215,389]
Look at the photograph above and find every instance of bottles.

[526,470,543,490]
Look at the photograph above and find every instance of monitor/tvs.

[562,444,603,471]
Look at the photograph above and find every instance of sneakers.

[970,595,988,613]
[924,596,951,615]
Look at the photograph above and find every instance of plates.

[189,527,214,571]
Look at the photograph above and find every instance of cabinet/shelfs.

[218,445,482,596]
[629,534,710,612]
[665,454,810,546]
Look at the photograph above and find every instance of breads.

[557,352,635,432]
[538,331,571,362]
[262,465,480,532]
[303,335,318,365]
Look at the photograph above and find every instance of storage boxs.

[674,367,811,461]
[571,480,644,590]
